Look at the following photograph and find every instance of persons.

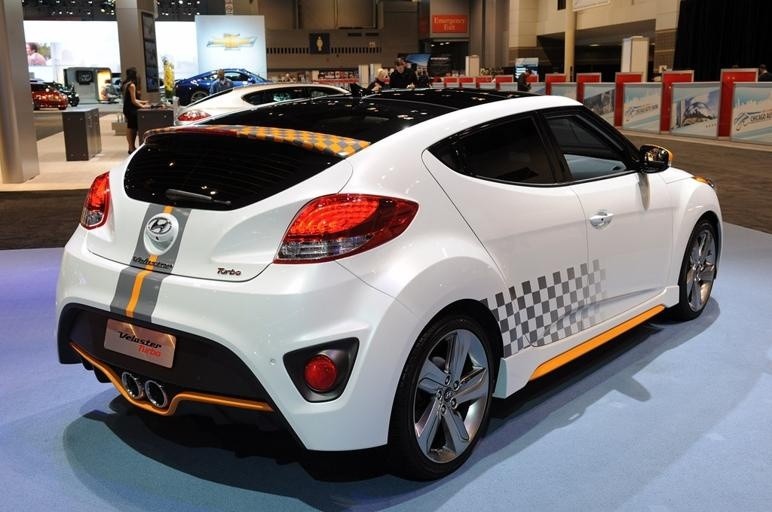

[518,69,532,92]
[162,55,175,106]
[209,69,233,95]
[101,80,120,104]
[122,67,151,154]
[758,64,772,81]
[416,68,430,89]
[26,43,47,65]
[348,58,417,94]
